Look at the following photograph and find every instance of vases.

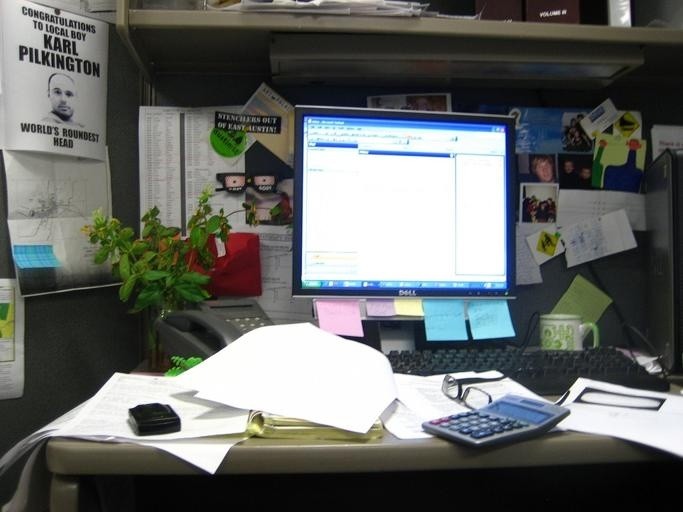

[142,293,191,372]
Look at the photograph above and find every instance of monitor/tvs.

[291,103,517,299]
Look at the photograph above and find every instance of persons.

[41,72,83,127]
[522,113,591,223]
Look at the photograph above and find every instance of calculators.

[422,394,571,448]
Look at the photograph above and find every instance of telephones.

[153,298,275,361]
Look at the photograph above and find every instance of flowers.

[82,182,246,359]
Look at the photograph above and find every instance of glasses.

[442,363,570,410]
[216,173,279,193]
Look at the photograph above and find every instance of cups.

[537,313,600,352]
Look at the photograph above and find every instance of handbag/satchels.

[183,232,261,296]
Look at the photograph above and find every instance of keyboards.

[384,348,670,396]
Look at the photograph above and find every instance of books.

[246,410,385,441]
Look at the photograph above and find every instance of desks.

[43,362,680,508]
